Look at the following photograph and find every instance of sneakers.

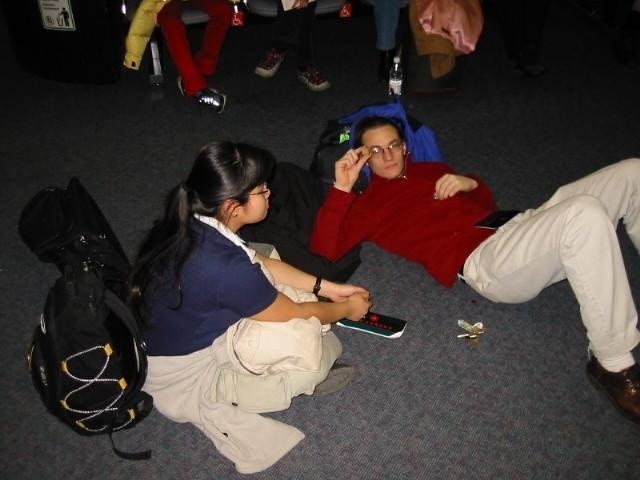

[521,42,543,76]
[177,75,187,98]
[191,87,226,115]
[297,63,332,92]
[437,59,463,91]
[378,49,398,85]
[509,57,527,79]
[255,47,283,78]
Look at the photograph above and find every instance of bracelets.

[312,276,323,297]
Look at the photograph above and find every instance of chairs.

[249,0,347,18]
[121,0,236,85]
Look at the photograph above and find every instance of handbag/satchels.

[237,160,362,284]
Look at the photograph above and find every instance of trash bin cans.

[7,0,148,86]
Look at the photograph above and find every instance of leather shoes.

[314,359,355,399]
[586,351,639,427]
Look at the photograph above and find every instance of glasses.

[368,138,404,158]
[249,182,270,197]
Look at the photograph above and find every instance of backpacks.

[314,118,369,204]
[18,174,154,460]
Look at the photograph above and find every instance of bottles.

[388,55,403,98]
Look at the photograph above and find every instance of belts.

[459,264,466,284]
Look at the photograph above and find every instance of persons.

[312,113,639,422]
[122,136,377,420]
[151,0,400,114]
